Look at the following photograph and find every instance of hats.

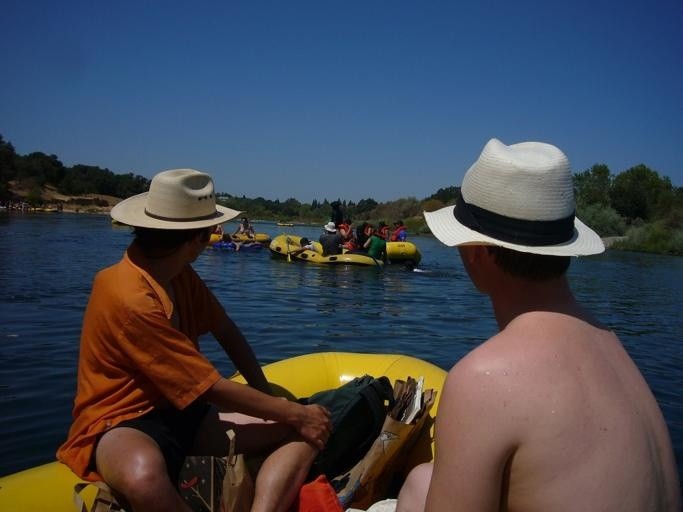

[423,138,604,256]
[109,169,247,230]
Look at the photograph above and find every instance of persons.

[212,224,223,237]
[287,200,408,261]
[230,216,256,238]
[392,139,682,510]
[55,166,337,510]
[216,233,262,253]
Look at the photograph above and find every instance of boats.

[17,207,57,212]
[206,233,270,246]
[0,351,449,511]
[269,232,383,267]
[110,219,132,226]
[276,222,294,226]
[340,240,421,268]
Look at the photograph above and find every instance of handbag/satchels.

[175,454,254,512]
[291,375,393,480]
